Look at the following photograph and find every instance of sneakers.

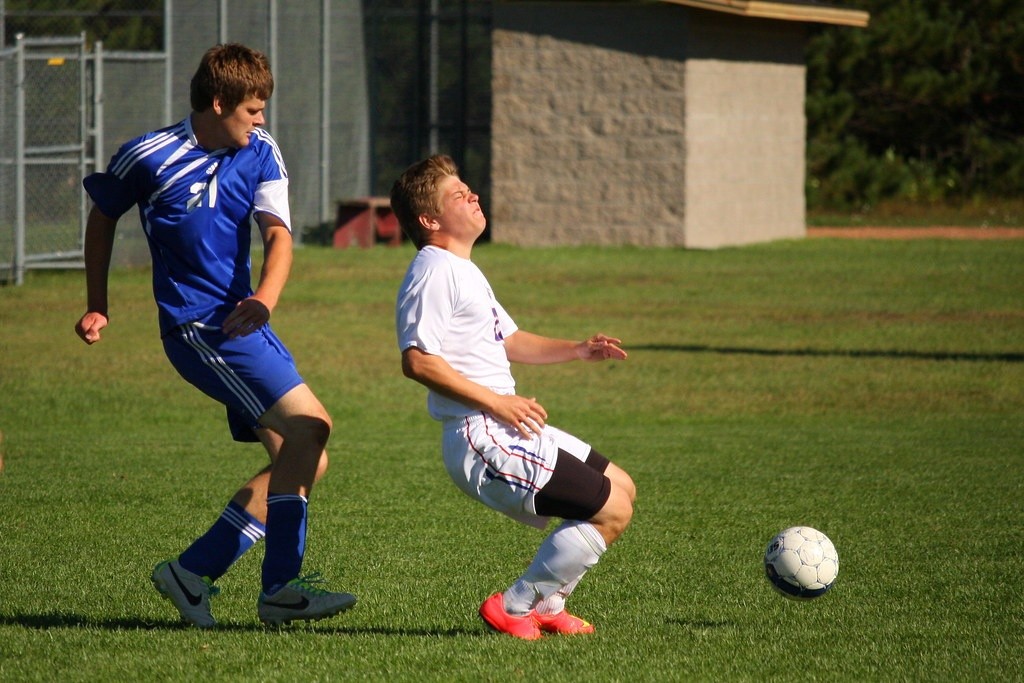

[530,608,594,634]
[150,557,220,627]
[257,572,357,626]
[479,592,545,640]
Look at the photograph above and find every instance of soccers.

[763,525,840,602]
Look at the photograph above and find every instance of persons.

[388,152,638,643]
[74,40,359,631]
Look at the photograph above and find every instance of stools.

[331,196,402,247]
